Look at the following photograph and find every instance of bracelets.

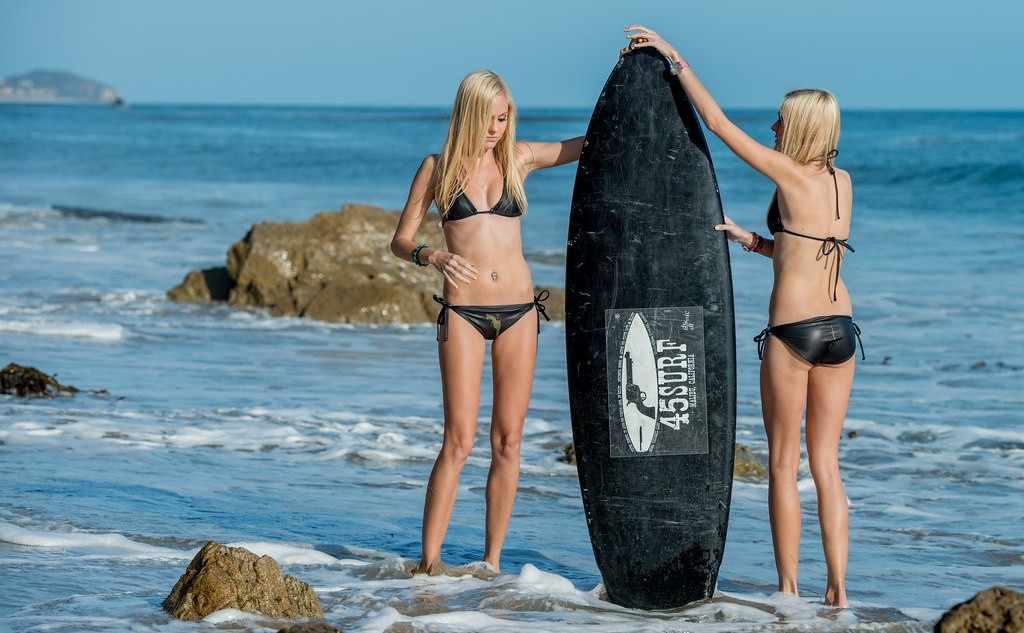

[742,231,764,254]
[411,242,429,267]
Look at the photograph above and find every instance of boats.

[564,46,737,610]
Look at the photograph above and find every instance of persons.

[624,23,865,608]
[390,37,648,577]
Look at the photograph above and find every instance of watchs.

[669,58,688,76]
